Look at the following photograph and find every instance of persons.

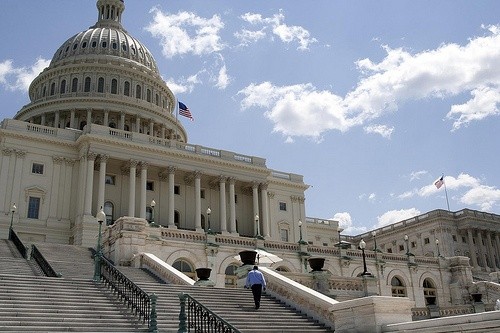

[246,266,266,309]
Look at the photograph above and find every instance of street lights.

[297,218,304,244]
[150,198,156,225]
[403,234,410,255]
[255,213,261,238]
[205,206,212,234]
[337,226,343,246]
[95,205,105,255]
[435,238,442,257]
[372,231,378,251]
[359,239,371,275]
[10,203,17,228]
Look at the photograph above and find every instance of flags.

[179,102,192,120]
[435,176,444,189]
